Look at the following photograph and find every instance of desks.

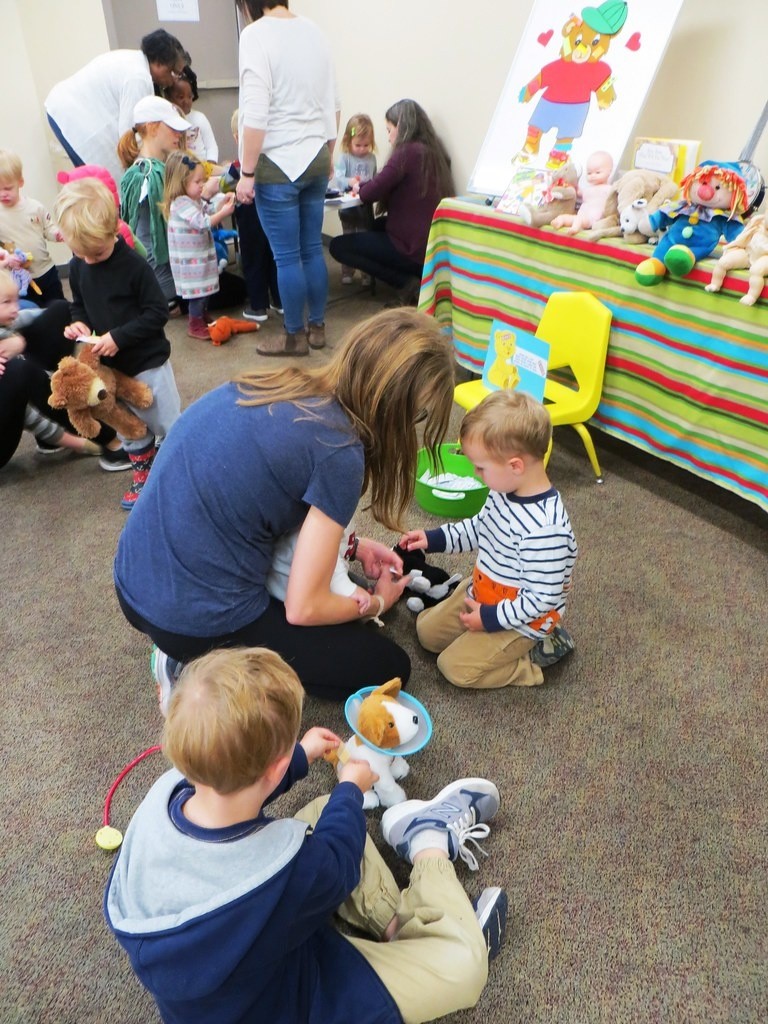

[231,193,377,296]
[416,194,768,513]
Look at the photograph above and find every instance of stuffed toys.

[211,227,238,274]
[582,158,765,287]
[520,160,579,227]
[47,342,155,441]
[390,542,464,614]
[207,316,260,346]
[323,678,411,810]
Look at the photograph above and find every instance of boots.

[304,322,325,348]
[120,435,156,511]
[258,324,309,356]
[188,316,213,339]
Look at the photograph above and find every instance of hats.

[133,95,192,131]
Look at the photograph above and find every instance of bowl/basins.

[408,443,490,520]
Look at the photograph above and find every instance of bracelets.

[370,594,386,628]
[349,538,360,562]
[242,170,254,177]
[201,195,210,204]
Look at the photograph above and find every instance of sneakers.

[528,622,572,668]
[270,301,284,314]
[470,885,508,962]
[243,304,268,322]
[381,778,500,872]
[149,642,187,718]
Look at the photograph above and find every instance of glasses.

[161,63,185,81]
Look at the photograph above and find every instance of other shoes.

[98,436,165,471]
[34,433,66,453]
[341,273,353,284]
[360,274,371,286]
[383,278,422,309]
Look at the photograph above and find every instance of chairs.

[453,290,614,482]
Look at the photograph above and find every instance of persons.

[44,26,184,210]
[0,148,138,473]
[112,305,457,724]
[227,0,455,355]
[104,648,510,1023]
[705,211,768,306]
[54,179,183,510]
[551,149,615,238]
[266,469,404,616]
[113,72,245,340]
[399,389,577,690]
